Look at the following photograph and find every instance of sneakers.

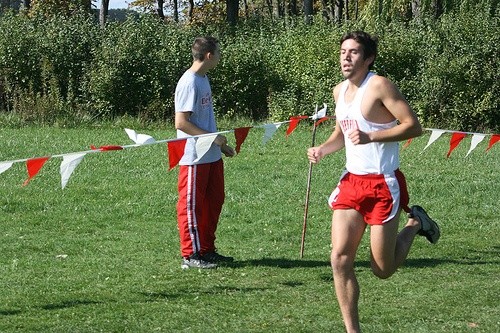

[181,253,217,269]
[202,251,234,262]
[407,205,440,244]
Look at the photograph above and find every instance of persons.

[173,35,235,269]
[305,30,441,333]
